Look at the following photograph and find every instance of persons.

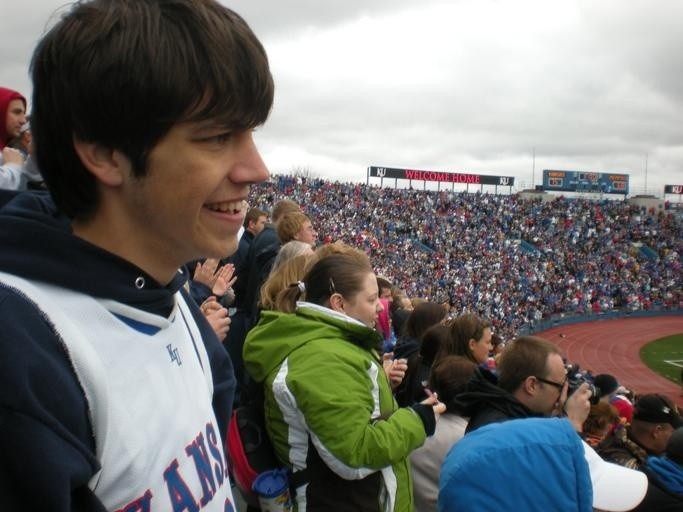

[181,210,682,512]
[0,85,47,192]
[14,115,33,159]
[0,0,276,512]
[247,173,683,344]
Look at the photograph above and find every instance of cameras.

[567,382,601,405]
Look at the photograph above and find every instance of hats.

[631,393,683,430]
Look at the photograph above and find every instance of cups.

[252,469,294,512]
